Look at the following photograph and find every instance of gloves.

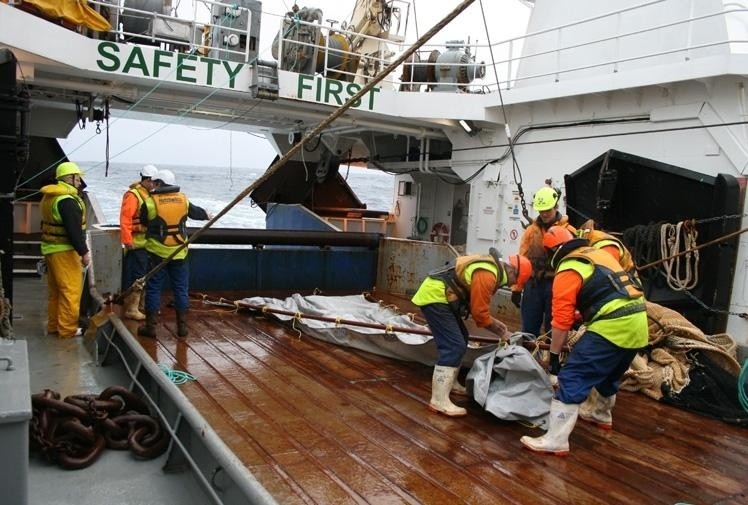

[548,351,561,376]
[511,291,521,308]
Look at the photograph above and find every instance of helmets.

[54,162,86,180]
[140,165,176,188]
[509,255,533,293]
[533,188,558,212]
[543,225,576,249]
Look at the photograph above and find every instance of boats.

[0,1,747,505]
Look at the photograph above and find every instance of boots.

[519,396,579,458]
[429,360,469,417]
[175,308,188,337]
[123,290,147,320]
[579,386,616,429]
[138,310,159,338]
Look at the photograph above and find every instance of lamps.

[457,119,483,138]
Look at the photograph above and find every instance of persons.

[120,165,158,320]
[562,218,646,300]
[39,162,90,336]
[140,166,214,338]
[511,187,577,343]
[411,248,533,417]
[520,224,649,457]
